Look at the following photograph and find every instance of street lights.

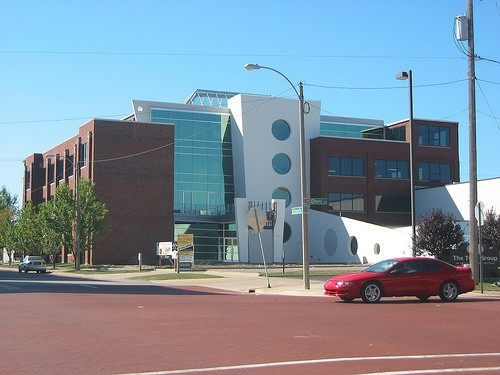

[45,145,77,270]
[395,69,417,258]
[244,62,311,290]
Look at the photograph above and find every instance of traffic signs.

[306,197,328,206]
[291,207,302,215]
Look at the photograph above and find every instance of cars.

[324,257,477,303]
[18,256,47,274]
